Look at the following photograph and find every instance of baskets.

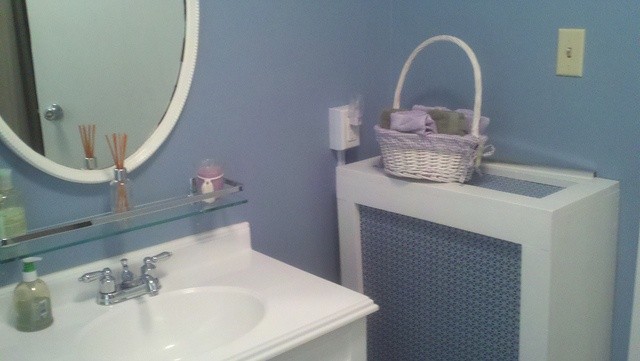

[373,34,496,183]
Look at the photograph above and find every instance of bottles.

[109,166,133,216]
[195,157,224,205]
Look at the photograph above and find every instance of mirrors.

[1,0,200,185]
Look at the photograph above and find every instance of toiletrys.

[0,167,28,242]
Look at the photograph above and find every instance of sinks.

[0,220,378,361]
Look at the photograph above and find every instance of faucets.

[118,257,159,298]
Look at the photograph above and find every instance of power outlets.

[329,106,361,151]
